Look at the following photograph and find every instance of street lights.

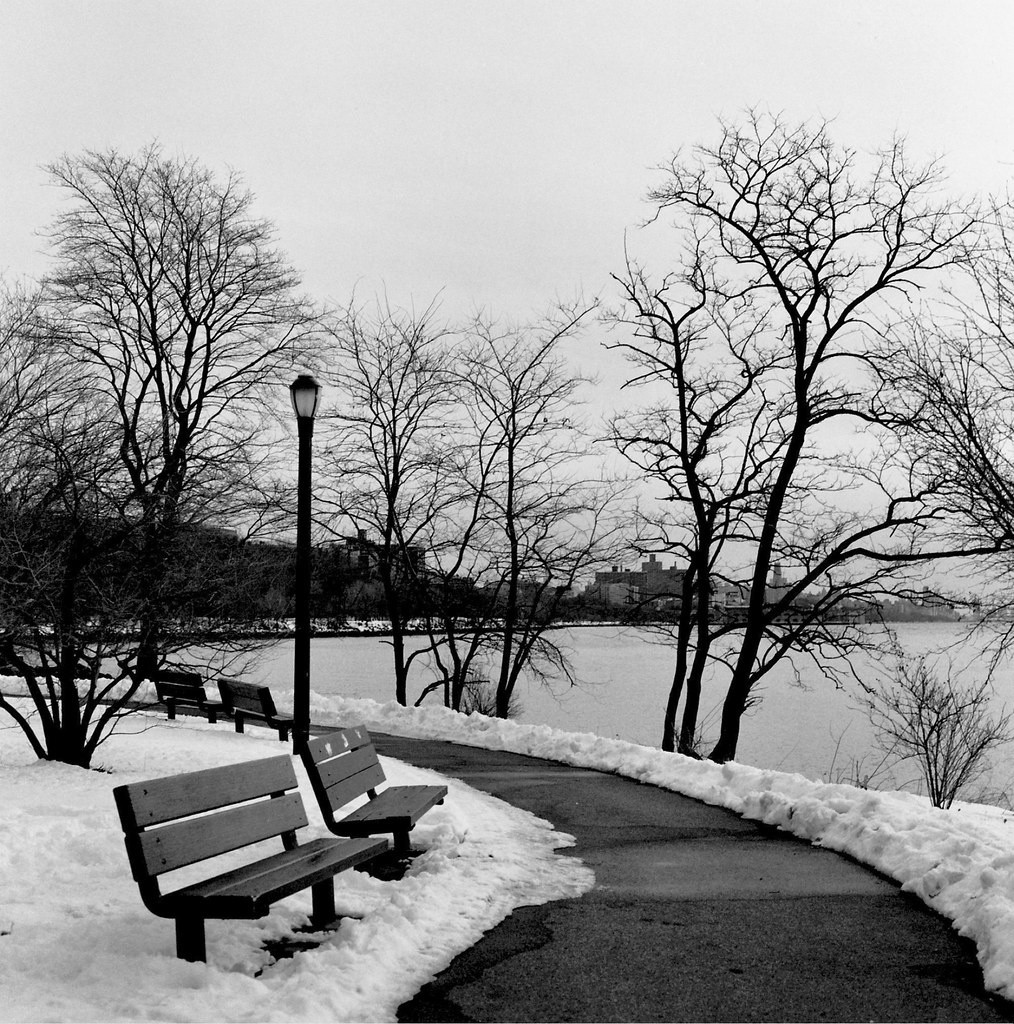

[286,374,325,756]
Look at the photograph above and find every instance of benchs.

[113,753,388,966]
[217,678,295,742]
[154,669,225,724]
[300,724,448,853]
